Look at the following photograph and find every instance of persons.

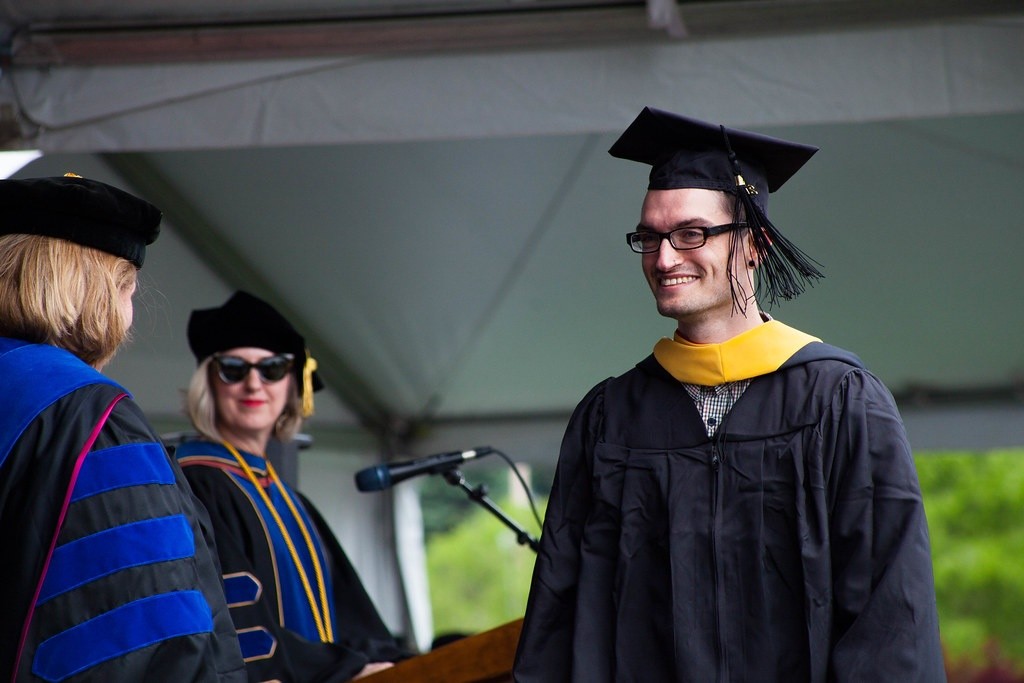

[512,109,945,683]
[173,291,418,683]
[0,173,246,682]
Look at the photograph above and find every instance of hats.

[0,151,163,271]
[608,105,827,319]
[186,290,325,418]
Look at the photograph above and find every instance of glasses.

[212,354,296,386]
[626,221,752,254]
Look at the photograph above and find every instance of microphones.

[355,446,493,493]
